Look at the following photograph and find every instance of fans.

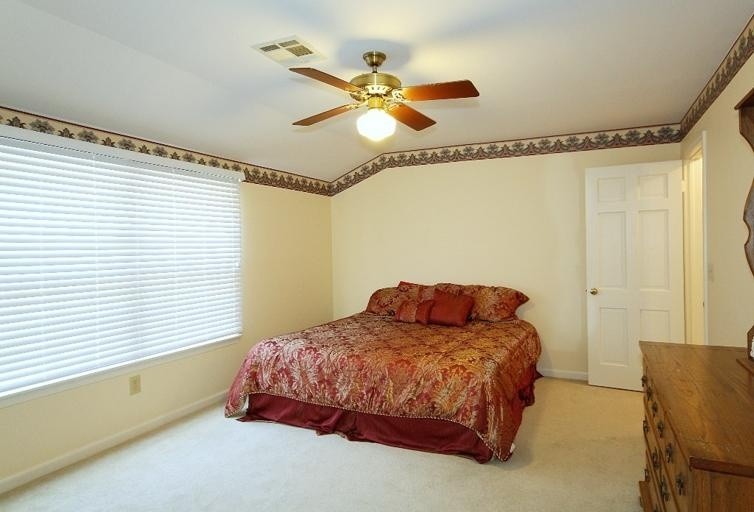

[283,53,482,144]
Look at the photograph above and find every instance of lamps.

[354,104,400,144]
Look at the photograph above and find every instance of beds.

[224,282,543,465]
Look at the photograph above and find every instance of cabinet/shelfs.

[637,342,754,512]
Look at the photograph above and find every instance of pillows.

[448,279,535,325]
[429,291,475,326]
[397,300,423,325]
[414,300,432,322]
[399,280,434,299]
[366,289,407,314]
